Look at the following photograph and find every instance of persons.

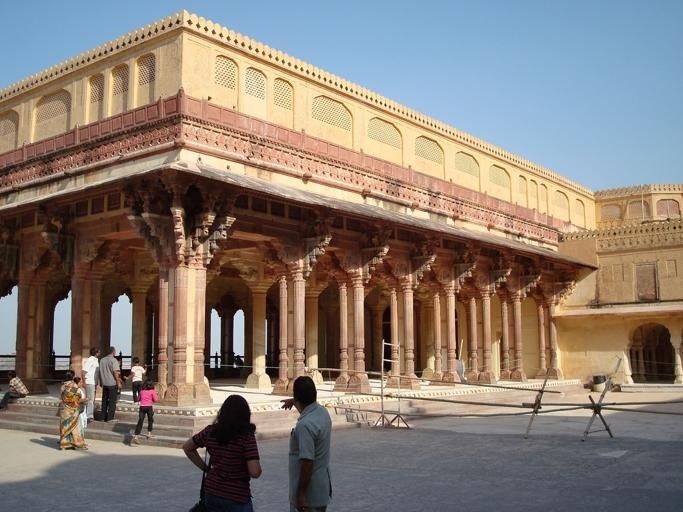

[98,346,122,422]
[180,393,262,512]
[123,356,147,404]
[81,346,100,422]
[56,369,88,452]
[232,354,243,369]
[132,378,161,444]
[279,376,334,511]
[0,368,30,411]
[72,376,88,441]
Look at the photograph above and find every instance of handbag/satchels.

[190,494,204,512]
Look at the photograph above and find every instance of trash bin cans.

[593,376,606,392]
